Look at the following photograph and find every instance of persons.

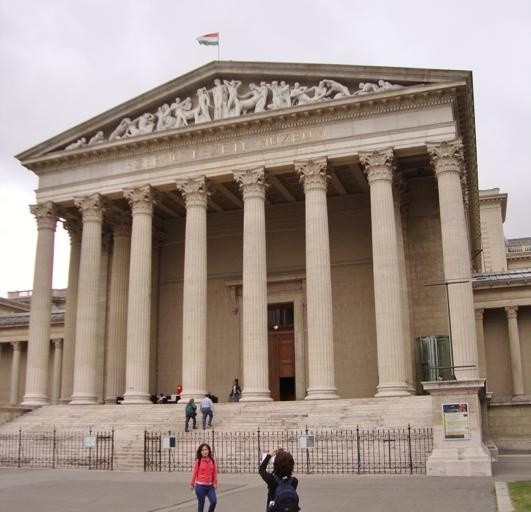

[189,442,219,512]
[156,392,167,404]
[228,378,242,402]
[259,446,302,511]
[184,397,198,432]
[200,392,215,430]
[65,78,407,152]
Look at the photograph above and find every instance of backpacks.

[270,473,300,511]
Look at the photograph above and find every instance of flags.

[196,32,220,47]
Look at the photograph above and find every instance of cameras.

[276,448,284,453]
